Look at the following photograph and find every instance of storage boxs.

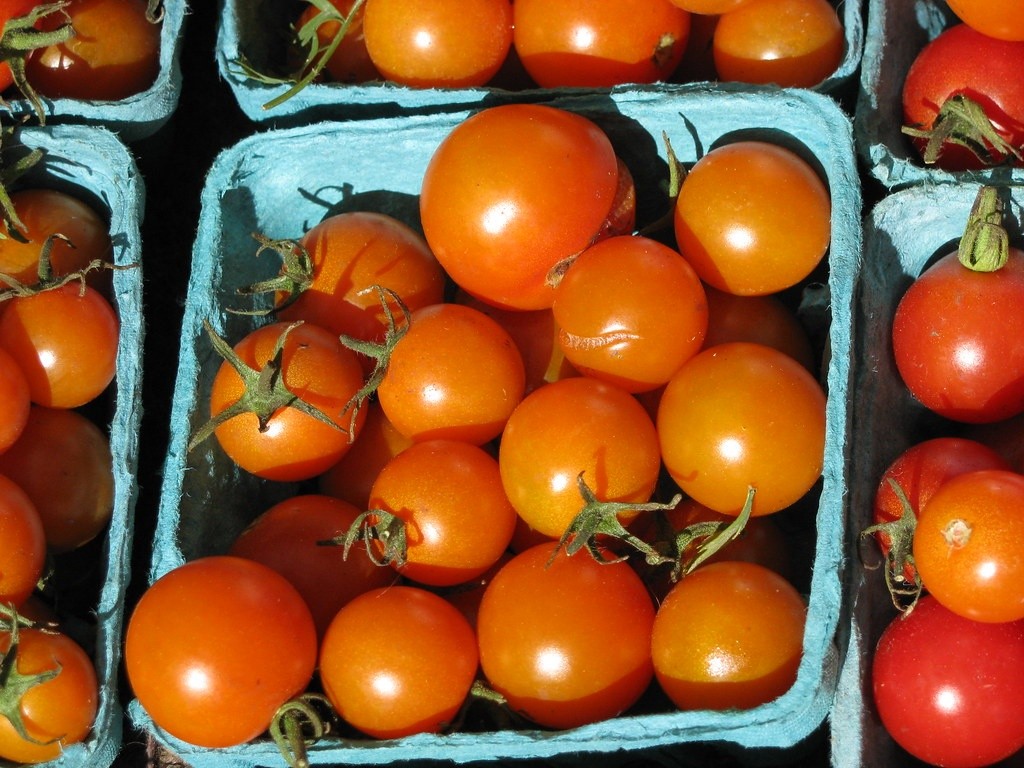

[2,0,1024,768]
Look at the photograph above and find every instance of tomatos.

[0,0,1024,768]
